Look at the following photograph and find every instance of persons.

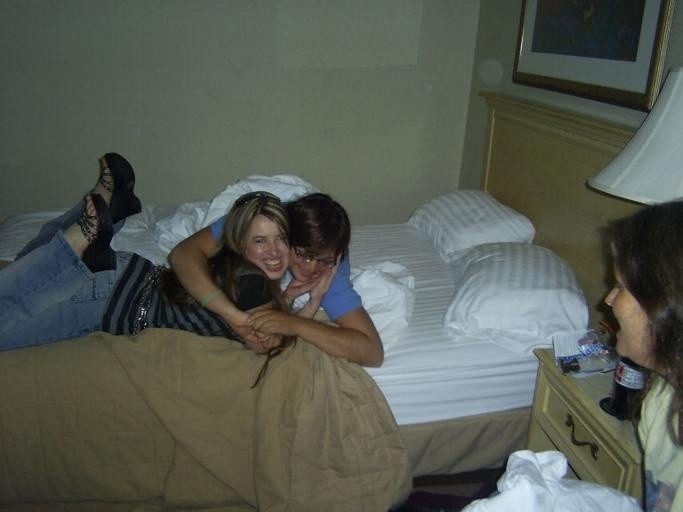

[163,192,385,368]
[0,153,342,353]
[604,196,683,512]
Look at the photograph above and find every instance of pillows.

[445,244,591,334]
[406,189,535,261]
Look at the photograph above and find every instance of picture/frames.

[511,0,677,110]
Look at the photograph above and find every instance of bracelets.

[200,287,223,308]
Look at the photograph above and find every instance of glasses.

[293,246,336,268]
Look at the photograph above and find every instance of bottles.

[605,357,650,420]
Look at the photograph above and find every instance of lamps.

[586,68,683,207]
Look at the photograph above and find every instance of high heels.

[99,152,142,223]
[76,194,116,273]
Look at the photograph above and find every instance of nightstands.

[524,349,644,505]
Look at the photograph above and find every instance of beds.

[0,90,650,511]
